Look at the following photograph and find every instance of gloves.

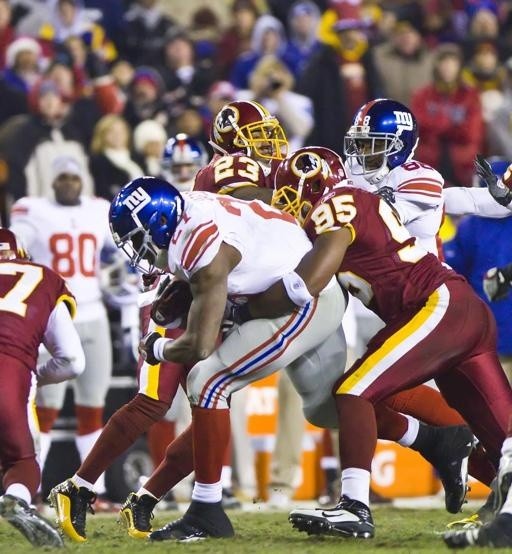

[138,331,160,366]
[150,276,193,326]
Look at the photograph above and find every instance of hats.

[50,157,83,187]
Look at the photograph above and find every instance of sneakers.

[289,423,512,548]
[0,476,240,547]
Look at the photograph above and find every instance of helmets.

[0,227,30,261]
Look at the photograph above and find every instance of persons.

[338,94,512,496]
[0,225,86,549]
[44,98,289,543]
[0,0,512,227]
[442,148,511,550]
[106,176,480,543]
[7,157,111,512]
[218,143,512,537]
